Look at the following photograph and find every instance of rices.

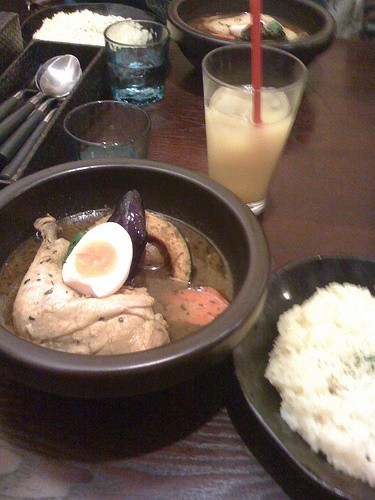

[32,9,154,47]
[264,282,375,489]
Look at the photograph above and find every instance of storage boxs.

[0,39,111,190]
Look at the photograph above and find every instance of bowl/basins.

[167,0,337,80]
[230,251,375,500]
[21,3,161,47]
[0,157,271,399]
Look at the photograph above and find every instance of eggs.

[63,222,133,296]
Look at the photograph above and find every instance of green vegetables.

[241,19,287,42]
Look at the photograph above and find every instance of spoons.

[0,54,82,179]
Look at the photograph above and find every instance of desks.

[0,36,375,500]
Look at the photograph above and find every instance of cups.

[63,100,152,161]
[103,20,170,106]
[200,43,310,216]
